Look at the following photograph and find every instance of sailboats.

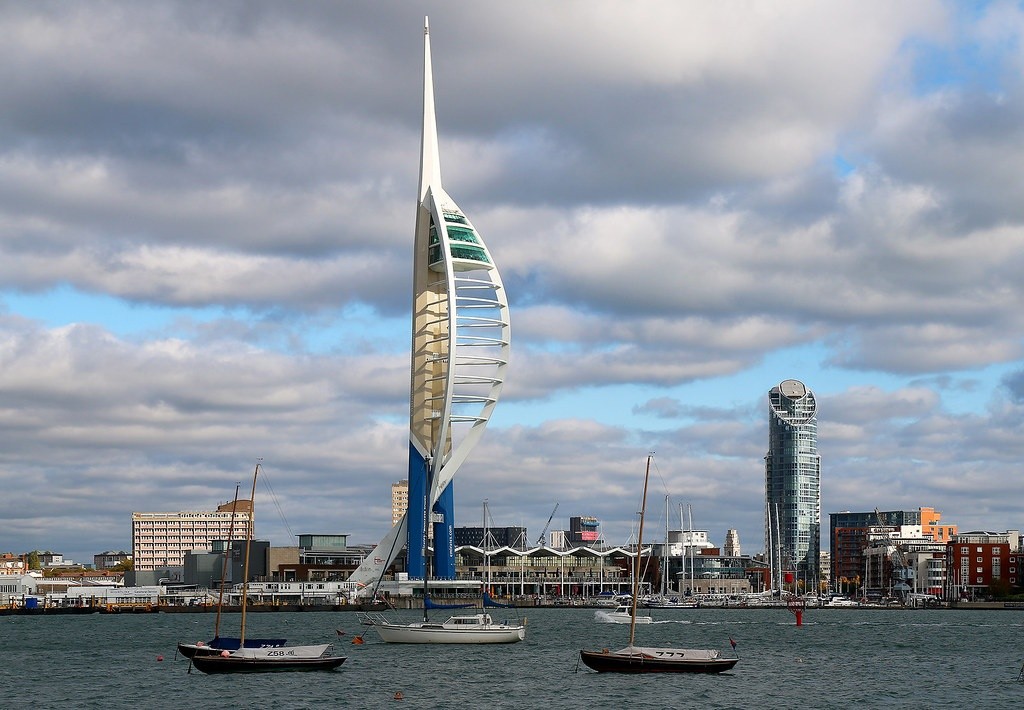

[170,461,346,674]
[641,493,865,607]
[576,451,741,674]
[354,456,528,643]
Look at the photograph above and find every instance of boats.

[597,605,652,624]
